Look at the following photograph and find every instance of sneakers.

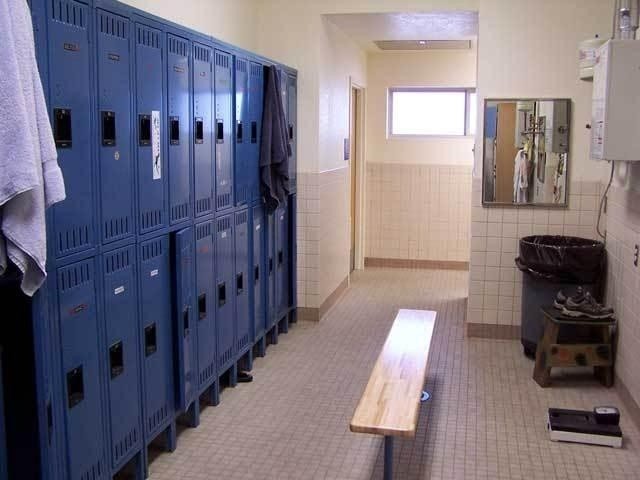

[562,288,616,321]
[552,287,605,310]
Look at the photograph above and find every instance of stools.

[532,307,616,388]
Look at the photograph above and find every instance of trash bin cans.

[514,235,607,359]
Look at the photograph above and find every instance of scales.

[546,406,623,448]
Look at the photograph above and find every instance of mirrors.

[482,98,570,207]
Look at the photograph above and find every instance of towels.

[513,150,529,203]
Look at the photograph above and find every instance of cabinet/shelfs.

[485,105,495,138]
[0,0,297,480]
[493,102,519,203]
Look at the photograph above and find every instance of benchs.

[350,309,437,480]
